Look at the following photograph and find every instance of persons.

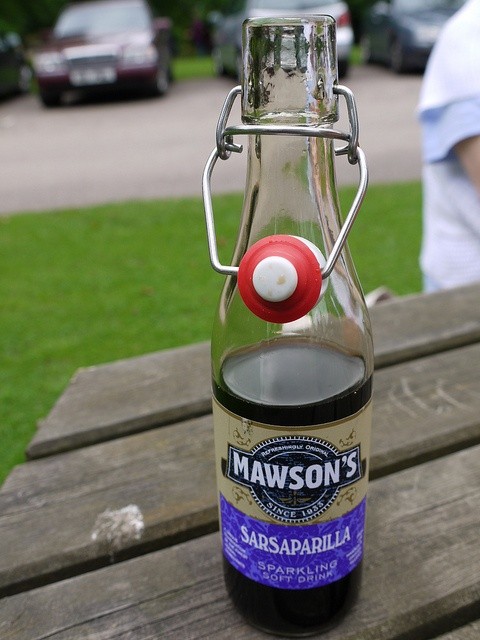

[418,1,480,294]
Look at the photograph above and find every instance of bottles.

[202,15,373,639]
